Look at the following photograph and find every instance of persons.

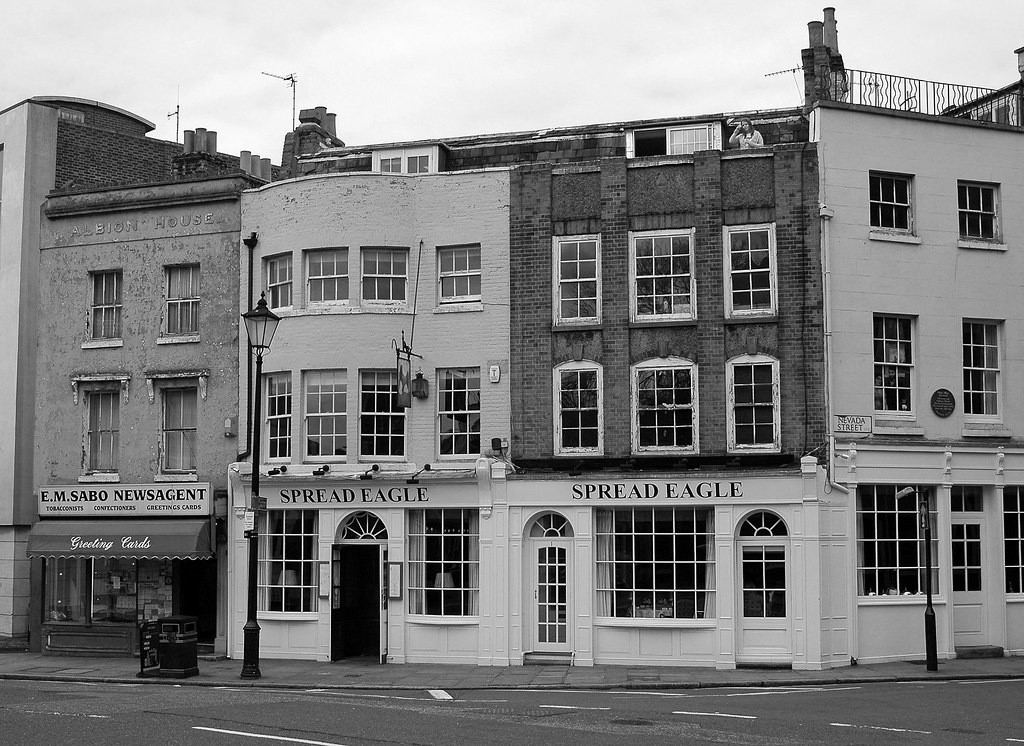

[729,117,764,149]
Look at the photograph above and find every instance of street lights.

[240,291,283,679]
[897,487,938,672]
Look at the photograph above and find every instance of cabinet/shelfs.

[98,593,136,620]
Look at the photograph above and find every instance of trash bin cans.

[156,616,199,678]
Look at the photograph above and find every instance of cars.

[625,595,673,618]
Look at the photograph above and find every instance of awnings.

[25,520,212,560]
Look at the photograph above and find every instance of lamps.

[267,466,287,477]
[407,463,432,486]
[314,463,331,477]
[357,463,382,482]
[409,368,430,399]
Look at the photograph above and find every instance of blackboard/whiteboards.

[141,629,159,669]
